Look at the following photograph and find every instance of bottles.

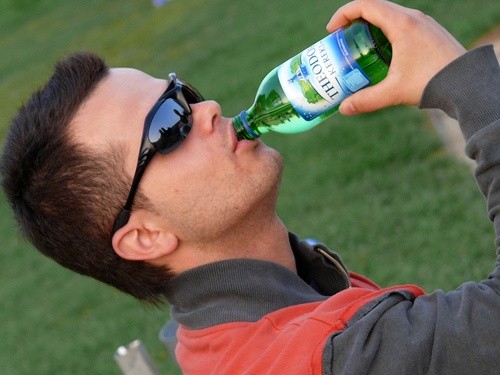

[231,18,394,142]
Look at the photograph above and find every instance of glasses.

[107,71,206,244]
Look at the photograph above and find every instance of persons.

[0,2,499,375]
